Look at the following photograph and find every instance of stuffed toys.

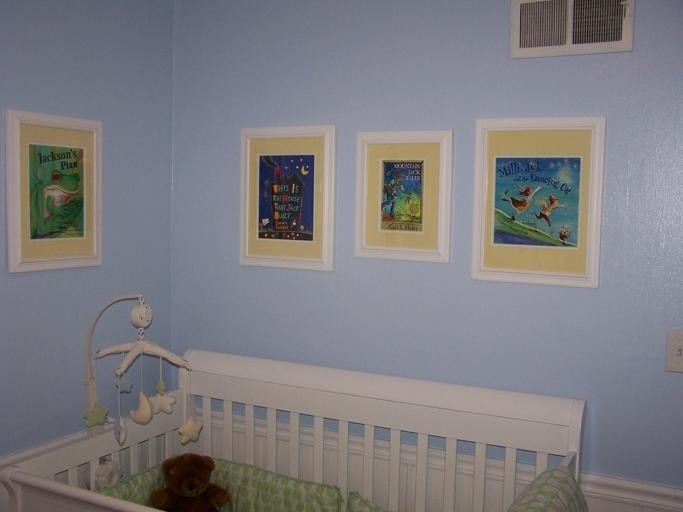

[147,453,234,512]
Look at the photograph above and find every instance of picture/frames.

[353,129,453,264]
[239,123,335,274]
[470,112,606,293]
[4,109,101,275]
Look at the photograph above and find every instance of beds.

[0,342,590,511]
